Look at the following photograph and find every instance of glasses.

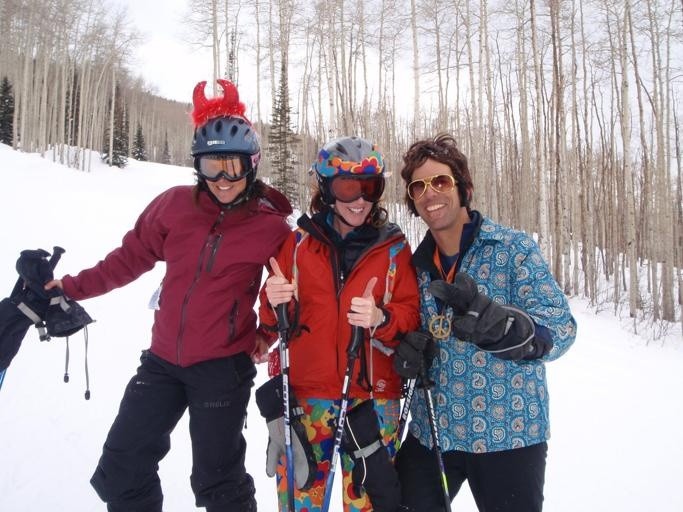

[407,174,463,201]
[311,168,386,203]
[194,150,260,183]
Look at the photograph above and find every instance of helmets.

[314,136,385,176]
[190,115,261,158]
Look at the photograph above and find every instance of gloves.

[0,249,94,375]
[254,373,317,492]
[392,329,436,379]
[427,272,553,364]
[341,399,402,510]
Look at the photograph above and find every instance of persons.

[41,117,294,512]
[258,136,421,512]
[392,129,576,512]
